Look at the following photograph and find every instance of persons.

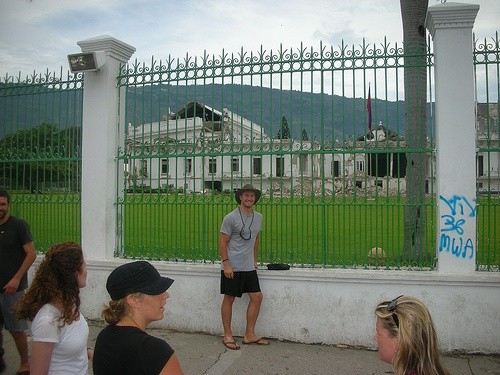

[373,295,450,375]
[0,189,36,375]
[12,242,93,375]
[92,261,183,375]
[219,184,270,350]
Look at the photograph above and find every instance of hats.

[106,261,175,300]
[235,184,261,206]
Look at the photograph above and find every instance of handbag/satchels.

[267,263,290,270]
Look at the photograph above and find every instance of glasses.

[387,295,403,329]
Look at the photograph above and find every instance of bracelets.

[223,259,228,262]
[87,347,93,350]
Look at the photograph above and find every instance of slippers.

[241,336,270,345]
[222,338,239,350]
